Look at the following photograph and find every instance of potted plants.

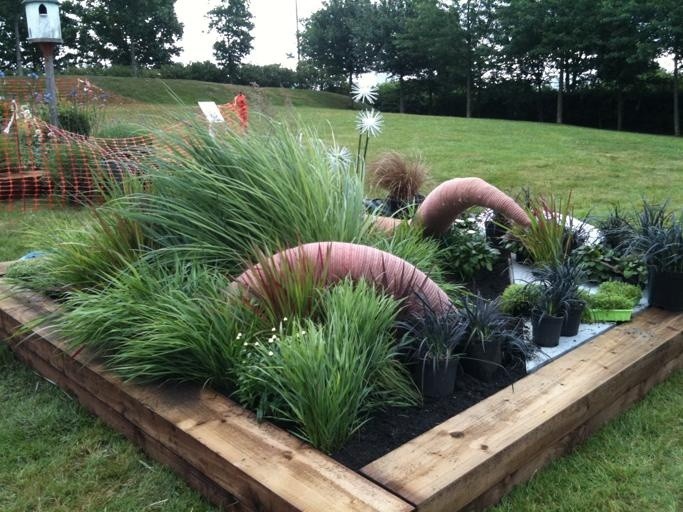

[366,151,683,397]
